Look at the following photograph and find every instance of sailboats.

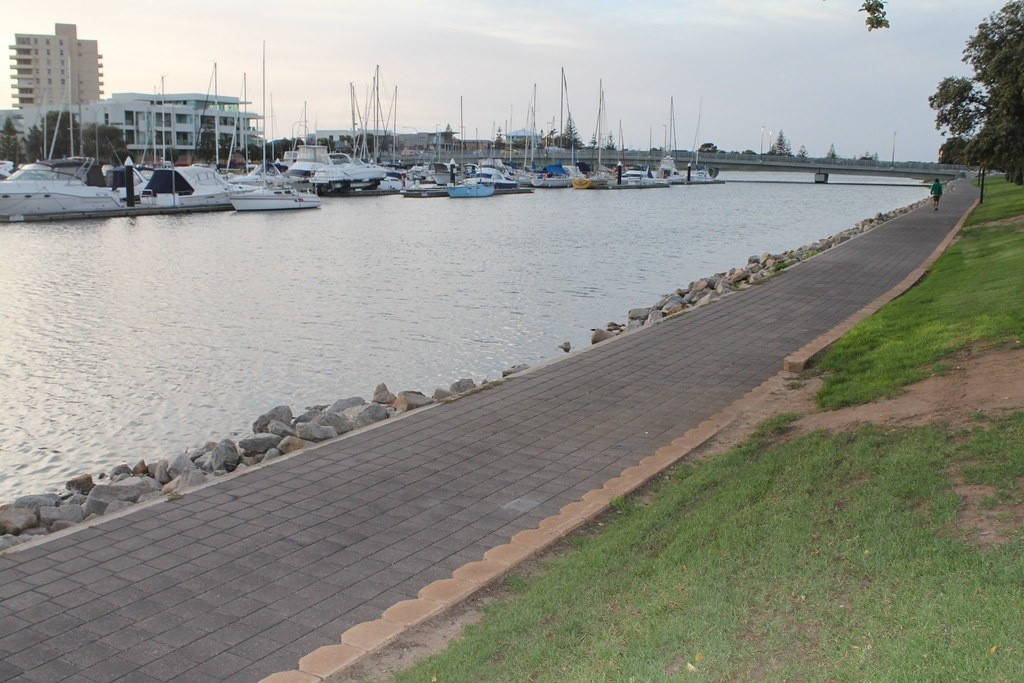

[668,97,726,185]
[228,38,322,211]
[0,54,678,197]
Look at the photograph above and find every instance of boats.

[140,165,255,208]
[0,159,124,217]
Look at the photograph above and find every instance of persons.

[930,179,942,211]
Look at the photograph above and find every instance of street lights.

[760,125,766,161]
[891,129,897,167]
